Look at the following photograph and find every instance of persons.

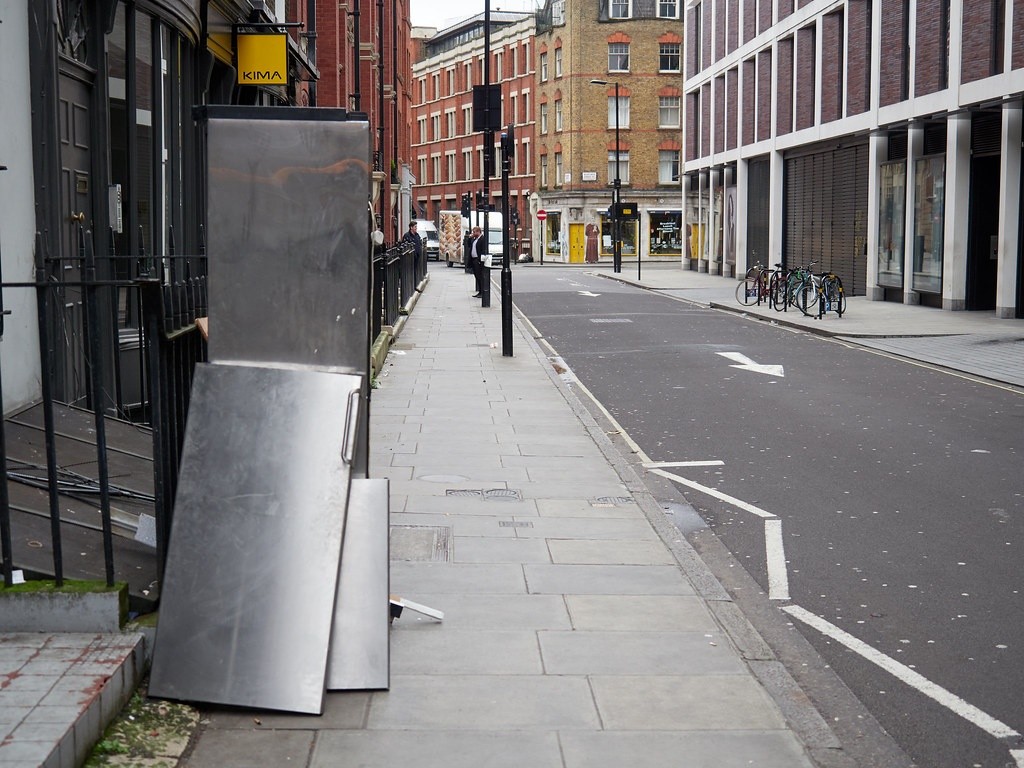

[402,222,422,292]
[467,226,485,297]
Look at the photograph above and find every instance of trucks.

[412,220,438,261]
[438,210,504,267]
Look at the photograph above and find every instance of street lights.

[588,79,622,273]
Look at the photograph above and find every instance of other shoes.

[472,293,480,297]
[477,293,482,297]
[415,288,422,293]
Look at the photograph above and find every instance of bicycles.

[735,250,846,317]
